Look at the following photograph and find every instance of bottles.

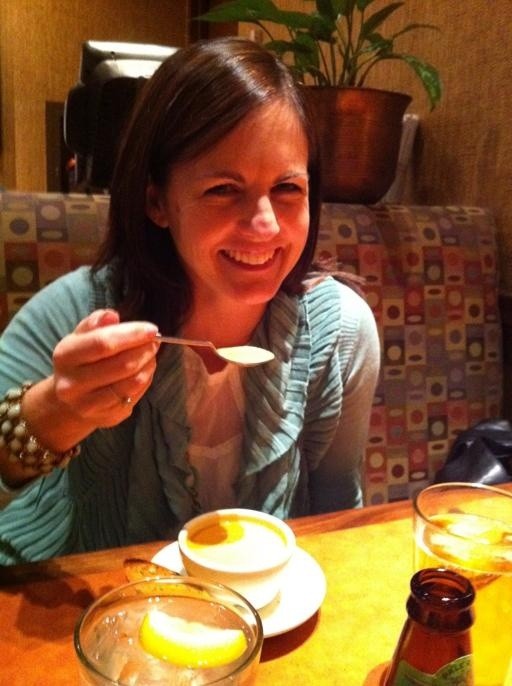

[379,566,475,686]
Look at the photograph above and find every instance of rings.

[104,379,137,406]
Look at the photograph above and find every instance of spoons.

[154,336,275,367]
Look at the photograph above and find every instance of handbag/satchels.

[434,416,512,485]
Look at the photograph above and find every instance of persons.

[0,35,383,570]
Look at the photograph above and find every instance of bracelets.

[0,382,84,476]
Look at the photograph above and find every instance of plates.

[144,539,326,641]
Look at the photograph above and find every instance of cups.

[74,578,264,686]
[408,481,512,595]
[177,507,297,611]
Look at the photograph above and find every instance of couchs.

[1,192,512,507]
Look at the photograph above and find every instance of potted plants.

[191,0,444,205]
